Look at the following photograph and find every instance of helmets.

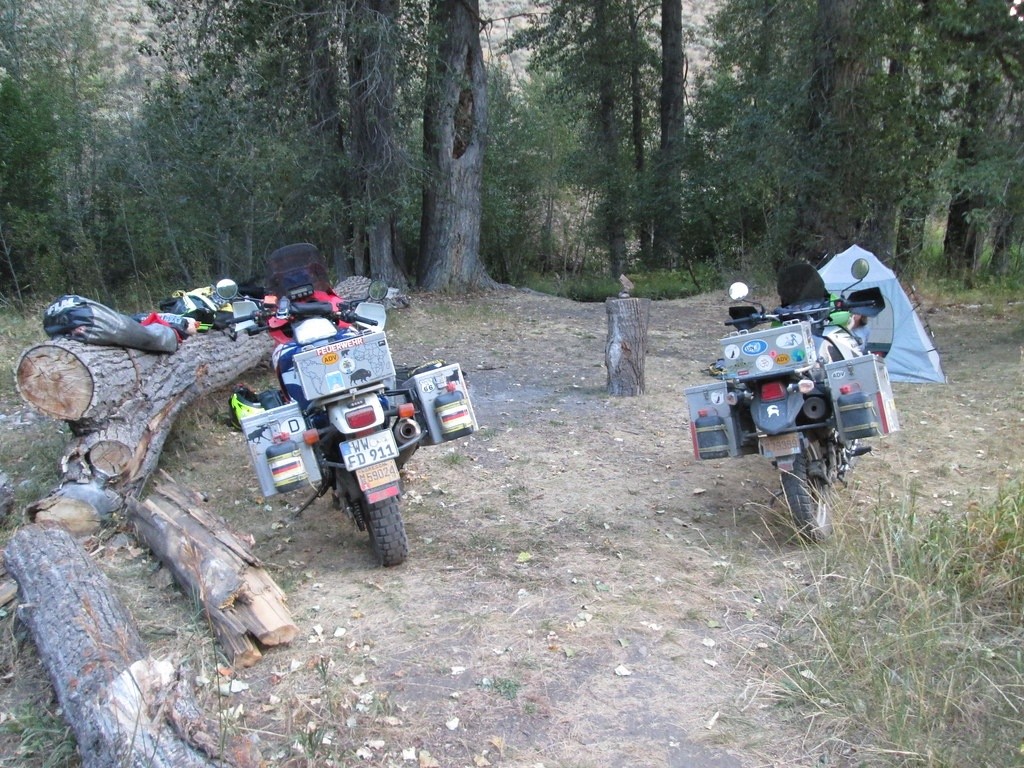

[227,388,266,433]
[776,263,830,308]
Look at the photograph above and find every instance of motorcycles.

[683,258,904,542]
[212,242,482,567]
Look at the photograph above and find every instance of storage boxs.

[291,330,396,401]
[240,401,323,498]
[824,354,900,440]
[401,363,479,447]
[684,382,759,459]
[721,321,817,381]
